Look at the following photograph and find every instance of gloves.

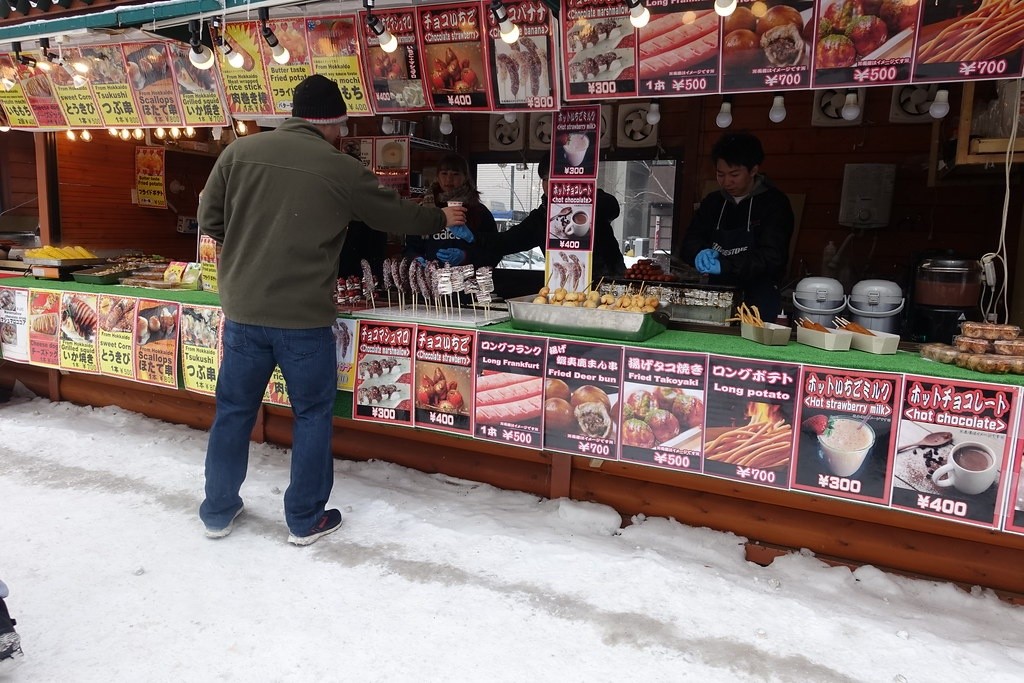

[695,248,721,275]
[436,248,464,266]
[449,223,473,243]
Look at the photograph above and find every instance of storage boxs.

[850,329,900,354]
[741,322,792,345]
[176,215,197,232]
[797,326,855,351]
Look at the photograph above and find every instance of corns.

[24,244,96,259]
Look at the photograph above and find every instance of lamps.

[929,83,950,120]
[382,114,394,136]
[236,120,248,136]
[55,35,88,88]
[211,16,244,68]
[769,92,786,122]
[39,37,64,66]
[491,0,520,44]
[842,88,860,122]
[258,7,290,65]
[504,112,518,122]
[646,97,661,126]
[12,42,36,67]
[362,0,398,54]
[340,122,349,138]
[714,0,738,18]
[628,1,650,28]
[439,112,453,135]
[187,19,215,70]
[717,94,732,128]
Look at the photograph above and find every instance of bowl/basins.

[379,120,421,136]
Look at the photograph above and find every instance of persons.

[338,152,387,291]
[194,75,466,545]
[436,152,627,277]
[675,130,795,327]
[400,155,503,268]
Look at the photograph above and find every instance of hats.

[292,74,349,124]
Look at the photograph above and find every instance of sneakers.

[288,509,343,546]
[205,501,244,538]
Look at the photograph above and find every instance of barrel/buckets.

[421,115,452,145]
[847,280,905,337]
[793,277,847,328]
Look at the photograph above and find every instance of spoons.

[550,206,572,222]
[898,432,952,453]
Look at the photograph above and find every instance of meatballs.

[546,377,614,441]
[621,386,708,450]
[817,0,922,69]
[722,5,814,69]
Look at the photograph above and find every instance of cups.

[565,210,590,237]
[447,201,463,207]
[563,132,589,166]
[816,414,875,478]
[931,441,996,494]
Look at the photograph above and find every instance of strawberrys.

[801,415,835,435]
[417,367,463,415]
[375,47,477,93]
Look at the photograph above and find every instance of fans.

[618,103,658,147]
[889,85,938,121]
[811,86,865,125]
[489,112,525,151]
[600,105,613,149]
[529,112,552,149]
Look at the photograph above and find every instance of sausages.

[553,251,582,290]
[635,9,719,78]
[801,321,831,333]
[361,258,441,299]
[624,260,676,282]
[472,372,543,424]
[839,322,876,336]
[496,35,551,98]
